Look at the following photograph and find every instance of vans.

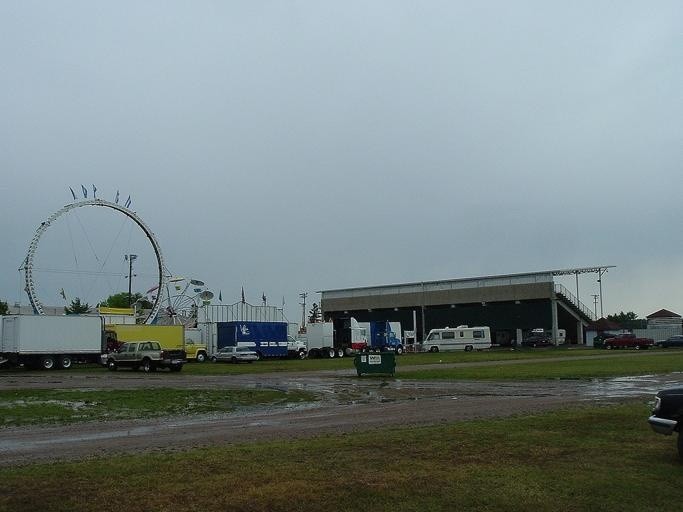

[422,325,492,353]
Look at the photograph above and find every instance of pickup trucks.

[604,333,654,350]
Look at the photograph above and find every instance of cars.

[648,388,683,465]
[656,335,683,348]
[593,333,617,347]
[524,328,567,349]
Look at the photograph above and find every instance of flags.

[92,183,97,198]
[125,195,131,208]
[114,191,121,205]
[68,186,76,200]
[81,185,90,198]
